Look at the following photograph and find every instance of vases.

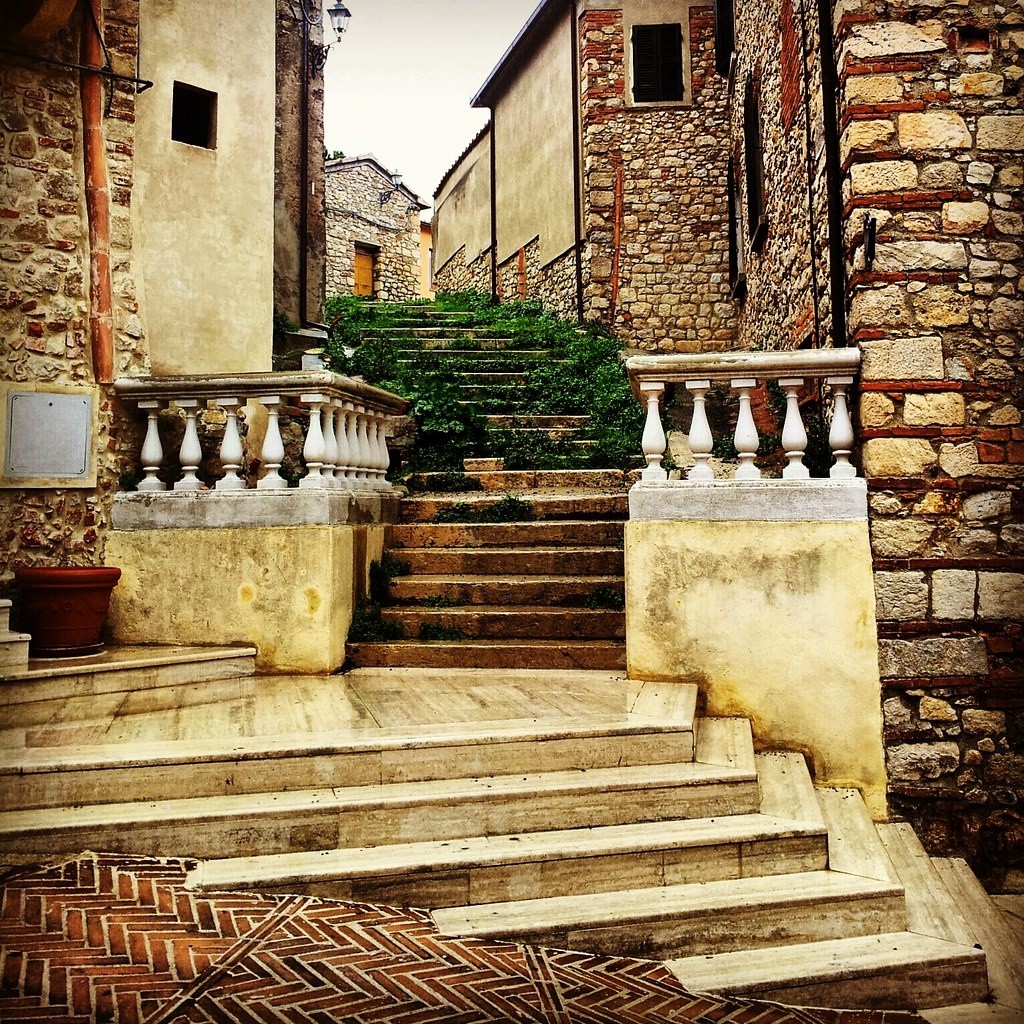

[15,567,121,648]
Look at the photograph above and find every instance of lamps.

[380,169,402,208]
[310,0,351,80]
